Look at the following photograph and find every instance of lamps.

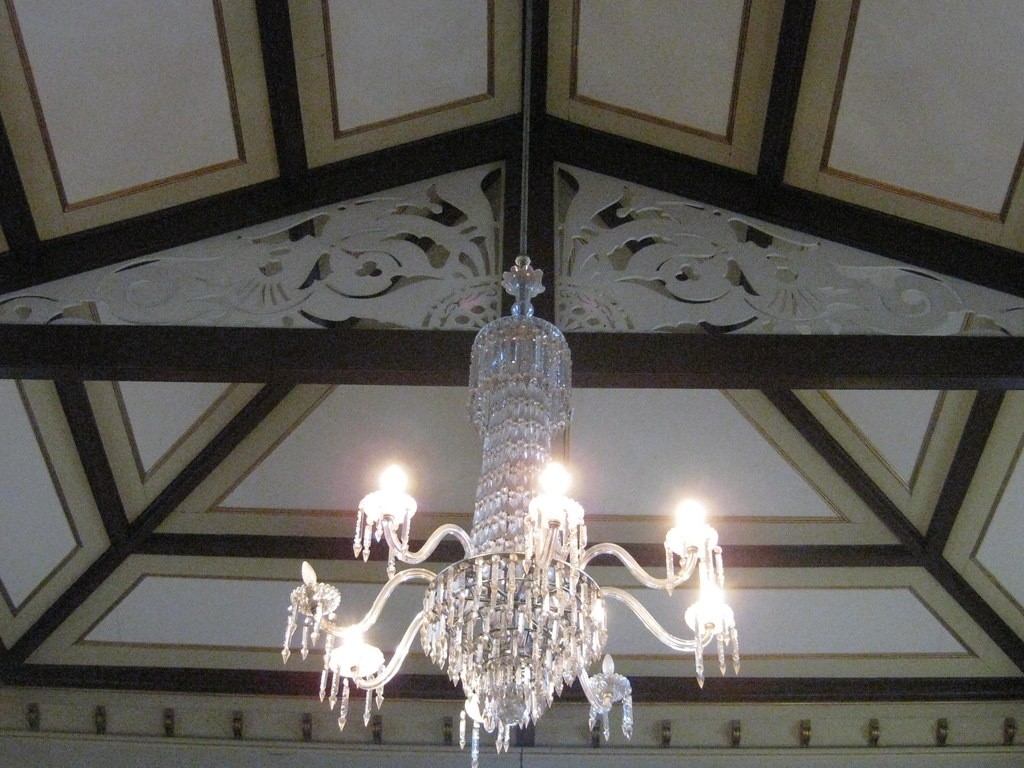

[280,0,744,768]
